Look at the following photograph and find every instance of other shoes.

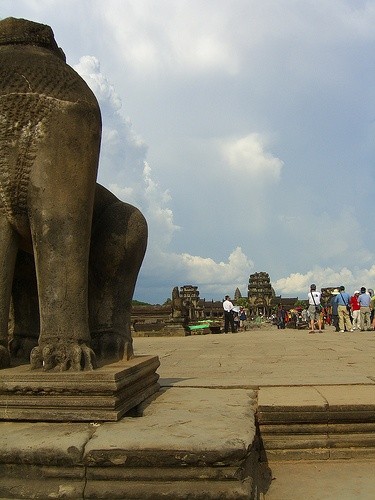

[349,329,353,332]
[319,331,322,333]
[309,331,314,333]
[232,330,238,333]
[339,330,344,332]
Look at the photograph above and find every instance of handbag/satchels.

[347,305,351,310]
[315,306,322,314]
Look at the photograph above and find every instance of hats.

[354,291,360,295]
[311,284,316,289]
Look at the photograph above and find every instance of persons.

[320,309,332,326]
[308,284,323,333]
[331,286,375,333]
[239,306,246,328]
[276,303,309,329]
[223,295,238,334]
